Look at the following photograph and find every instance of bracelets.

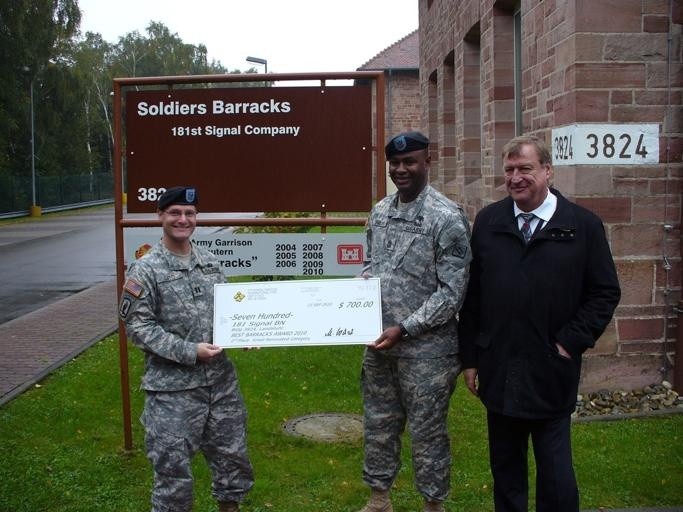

[398,322,408,339]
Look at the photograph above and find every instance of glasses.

[165,210,196,217]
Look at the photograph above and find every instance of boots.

[219,500,240,512]
[359,488,393,512]
[424,502,445,512]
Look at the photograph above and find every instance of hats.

[385,132,430,160]
[157,187,198,210]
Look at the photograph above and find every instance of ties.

[520,213,534,245]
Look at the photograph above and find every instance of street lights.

[29,55,58,218]
[245,56,268,87]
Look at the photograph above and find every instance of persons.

[453,131,621,512]
[119,186,255,511]
[357,129,473,512]
